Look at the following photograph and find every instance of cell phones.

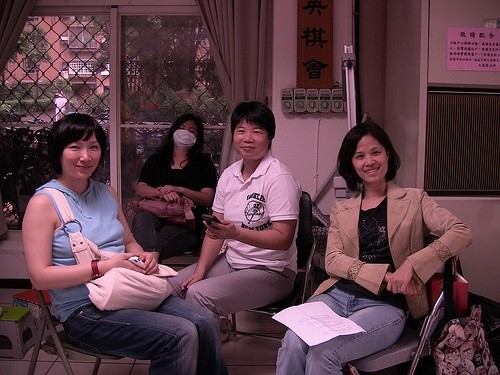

[202,214,222,225]
[128,256,145,269]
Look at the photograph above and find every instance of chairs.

[344,233,453,375]
[224,191,316,342]
[27,290,125,375]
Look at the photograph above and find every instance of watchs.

[91,260,103,280]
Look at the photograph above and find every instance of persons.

[20,115,230,375]
[166,102,300,318]
[130,112,220,266]
[274,120,474,374]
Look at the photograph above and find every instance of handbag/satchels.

[130,196,196,230]
[428,256,469,327]
[70,232,179,312]
[429,305,500,375]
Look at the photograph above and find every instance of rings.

[397,287,401,293]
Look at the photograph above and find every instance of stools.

[0,306,37,359]
[12,290,58,330]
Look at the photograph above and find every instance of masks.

[173,129,197,150]
